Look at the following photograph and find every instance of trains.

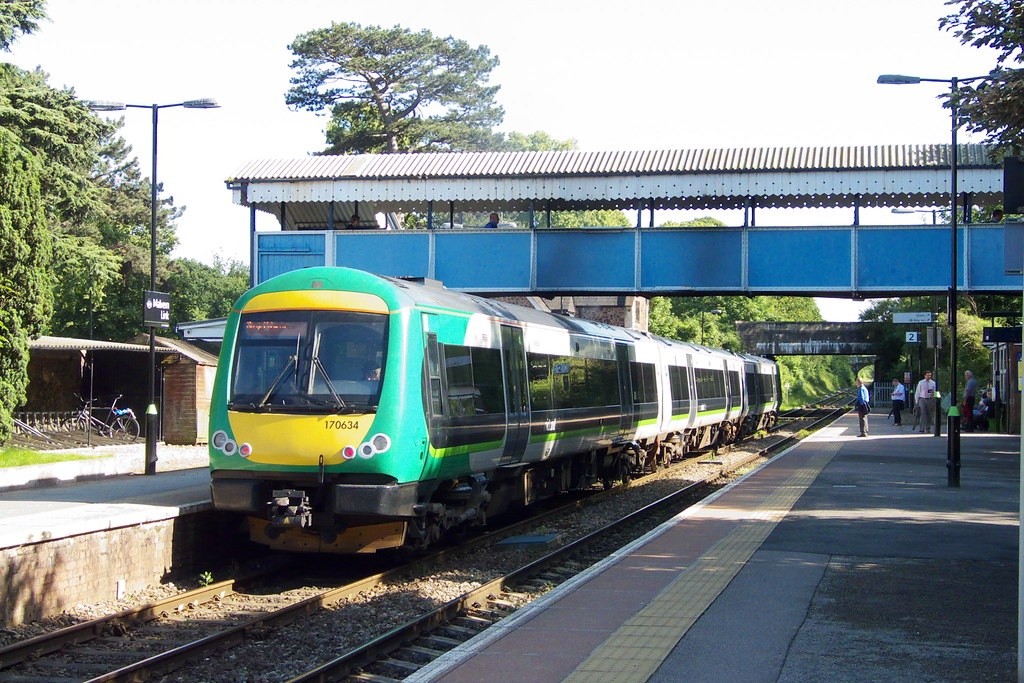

[205,263,784,564]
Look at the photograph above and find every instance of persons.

[960,389,995,431]
[855,378,870,437]
[914,368,936,434]
[960,371,976,432]
[890,378,906,426]
[359,360,382,381]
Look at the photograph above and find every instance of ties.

[925,379,929,398]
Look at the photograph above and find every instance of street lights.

[84,100,221,476]
[875,73,1006,489]
[888,206,953,436]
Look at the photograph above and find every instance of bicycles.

[60,392,140,444]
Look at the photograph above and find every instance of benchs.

[985,401,1008,434]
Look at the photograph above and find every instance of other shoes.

[920,427,931,434]
[857,433,870,437]
[892,423,902,426]
[963,427,973,432]
